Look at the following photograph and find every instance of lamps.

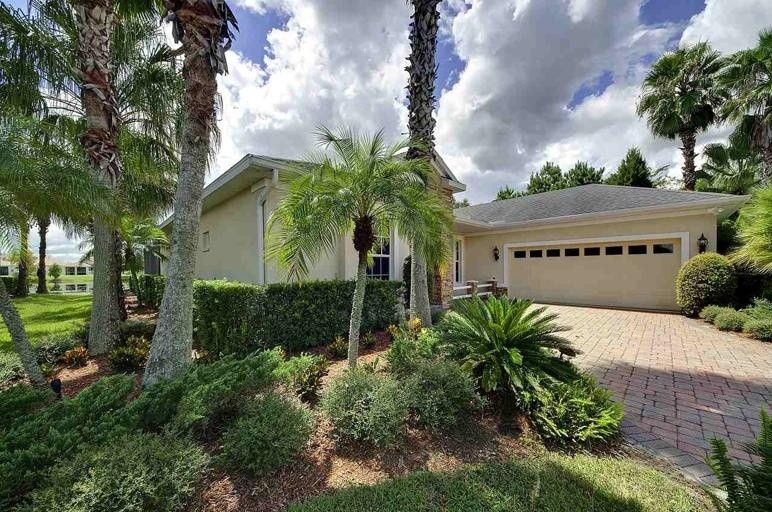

[493,245,499,261]
[697,232,708,254]
